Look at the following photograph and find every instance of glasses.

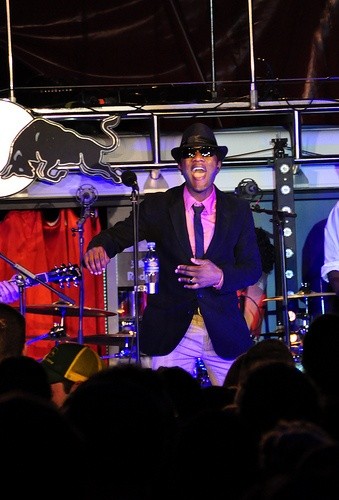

[183,146,215,157]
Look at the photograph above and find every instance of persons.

[321,200,339,296]
[0,274,339,500]
[84,124,262,383]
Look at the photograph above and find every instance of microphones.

[122,170,140,192]
[80,188,95,202]
[235,183,257,199]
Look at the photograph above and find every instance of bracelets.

[213,273,223,290]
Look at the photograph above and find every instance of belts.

[194,307,203,317]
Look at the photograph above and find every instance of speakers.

[44,343,103,383]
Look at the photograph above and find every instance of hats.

[40,342,103,382]
[171,123,229,160]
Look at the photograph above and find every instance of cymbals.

[25,335,69,343]
[11,301,117,317]
[260,291,336,302]
[68,333,136,347]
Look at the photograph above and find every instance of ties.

[191,204,205,259]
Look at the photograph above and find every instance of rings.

[189,278,193,284]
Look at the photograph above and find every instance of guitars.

[0,261,84,299]
